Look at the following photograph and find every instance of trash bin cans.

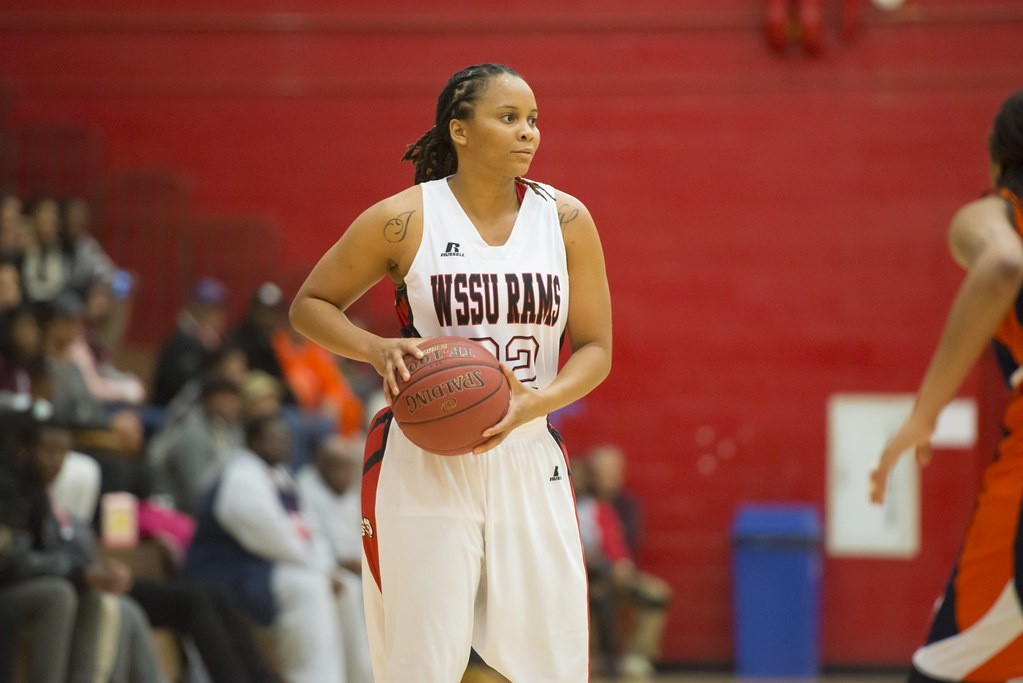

[731,502,821,683]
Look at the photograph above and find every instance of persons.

[0,184,673,683]
[287,58,613,683]
[867,89,1023,683]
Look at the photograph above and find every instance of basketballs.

[389,335,511,457]
